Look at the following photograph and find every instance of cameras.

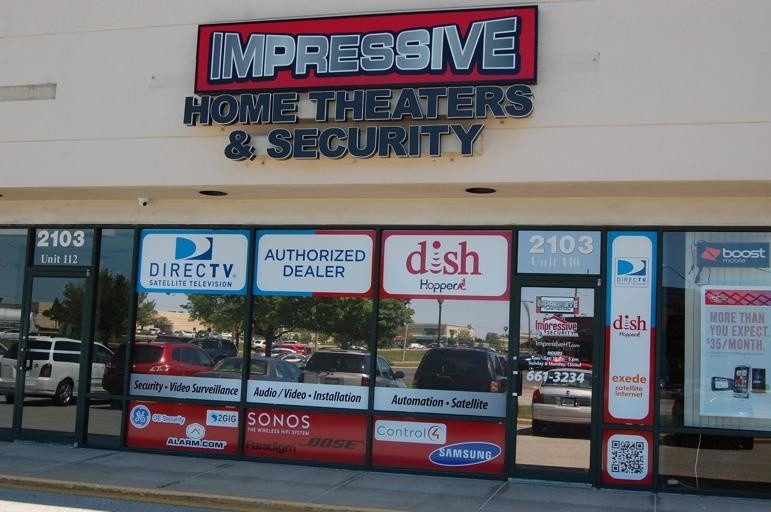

[711,377,734,391]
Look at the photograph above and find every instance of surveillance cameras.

[138,197,149,207]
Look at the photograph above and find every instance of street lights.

[438,298,445,349]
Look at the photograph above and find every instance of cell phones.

[752,368,766,393]
[734,365,752,397]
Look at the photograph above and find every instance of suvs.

[101,339,218,408]
[187,338,239,359]
[412,346,508,394]
[0,337,117,406]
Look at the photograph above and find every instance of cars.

[520,353,593,371]
[427,343,444,349]
[252,338,313,366]
[302,351,408,388]
[193,357,303,385]
[0,329,21,339]
[531,367,593,429]
[136,327,233,339]
[408,343,426,349]
